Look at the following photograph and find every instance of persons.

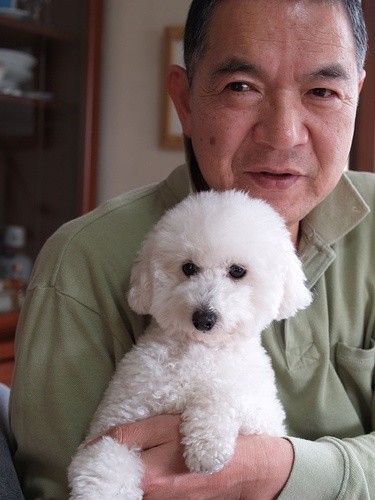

[9,0,375,500]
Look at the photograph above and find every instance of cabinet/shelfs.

[0,0,104,389]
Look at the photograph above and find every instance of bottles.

[0,223,33,313]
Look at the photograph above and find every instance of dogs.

[66,188,316,500]
